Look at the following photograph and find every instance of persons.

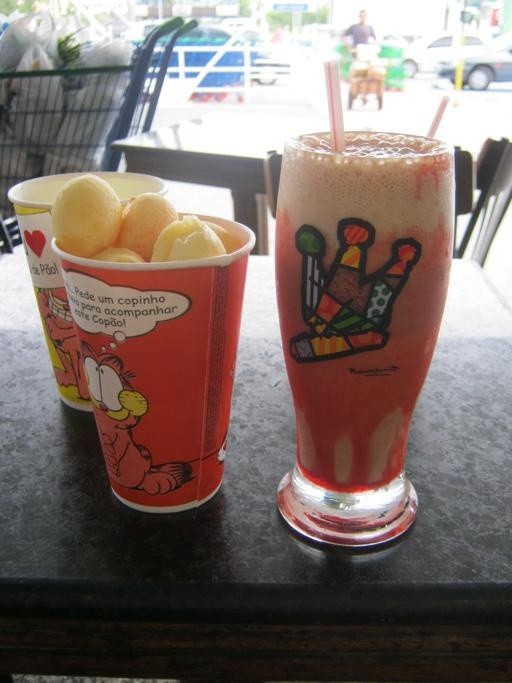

[340,8,377,60]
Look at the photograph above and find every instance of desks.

[0,251,511,682]
[110,117,284,256]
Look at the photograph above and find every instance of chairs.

[452,137,512,265]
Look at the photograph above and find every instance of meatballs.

[51,172,245,264]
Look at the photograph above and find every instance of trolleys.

[0,17,198,254]
[345,40,386,109]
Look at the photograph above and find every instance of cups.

[274,128,455,548]
[6,171,168,413]
[50,212,257,513]
